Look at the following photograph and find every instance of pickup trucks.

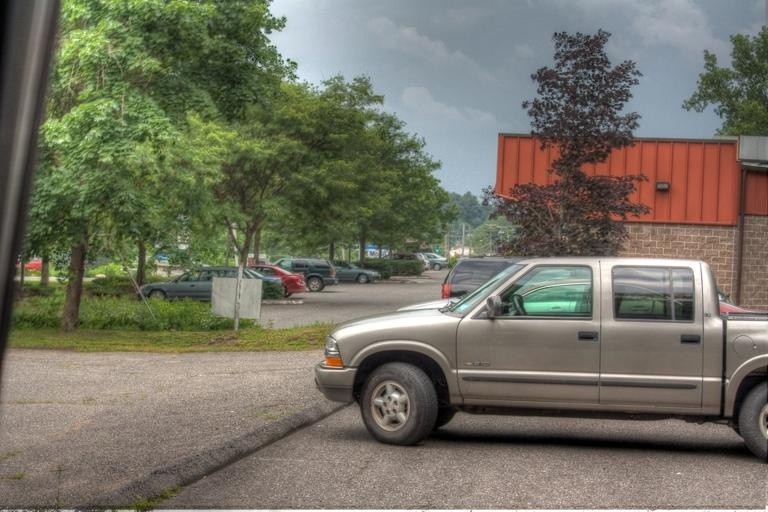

[314,255,767,461]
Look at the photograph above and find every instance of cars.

[332,260,380,285]
[14,255,42,273]
[253,264,305,298]
[381,251,449,273]
[217,264,284,300]
[136,266,264,306]
[152,253,169,264]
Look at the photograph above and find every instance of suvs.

[271,258,336,292]
